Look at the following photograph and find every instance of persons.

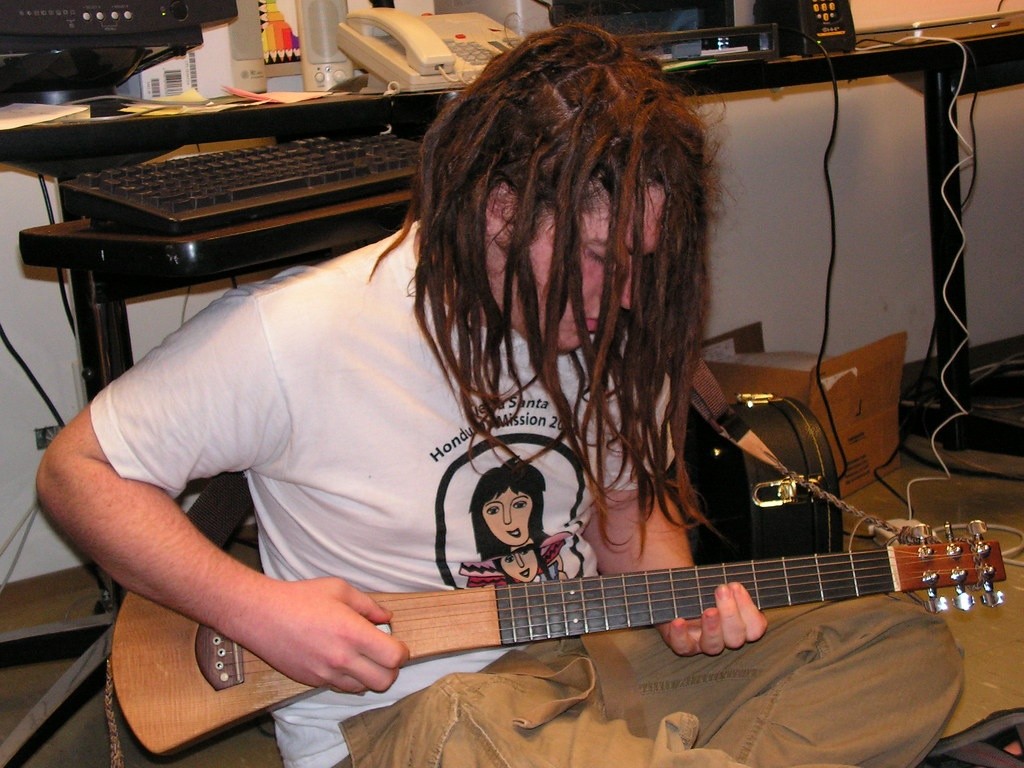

[35,21,963,768]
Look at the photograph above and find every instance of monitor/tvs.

[0,0,237,125]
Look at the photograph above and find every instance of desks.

[0,14,1024,451]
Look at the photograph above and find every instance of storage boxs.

[692,318,908,503]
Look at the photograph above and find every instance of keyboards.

[57,132,432,236]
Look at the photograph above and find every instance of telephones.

[334,6,526,93]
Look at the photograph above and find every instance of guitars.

[111,520,1010,759]
